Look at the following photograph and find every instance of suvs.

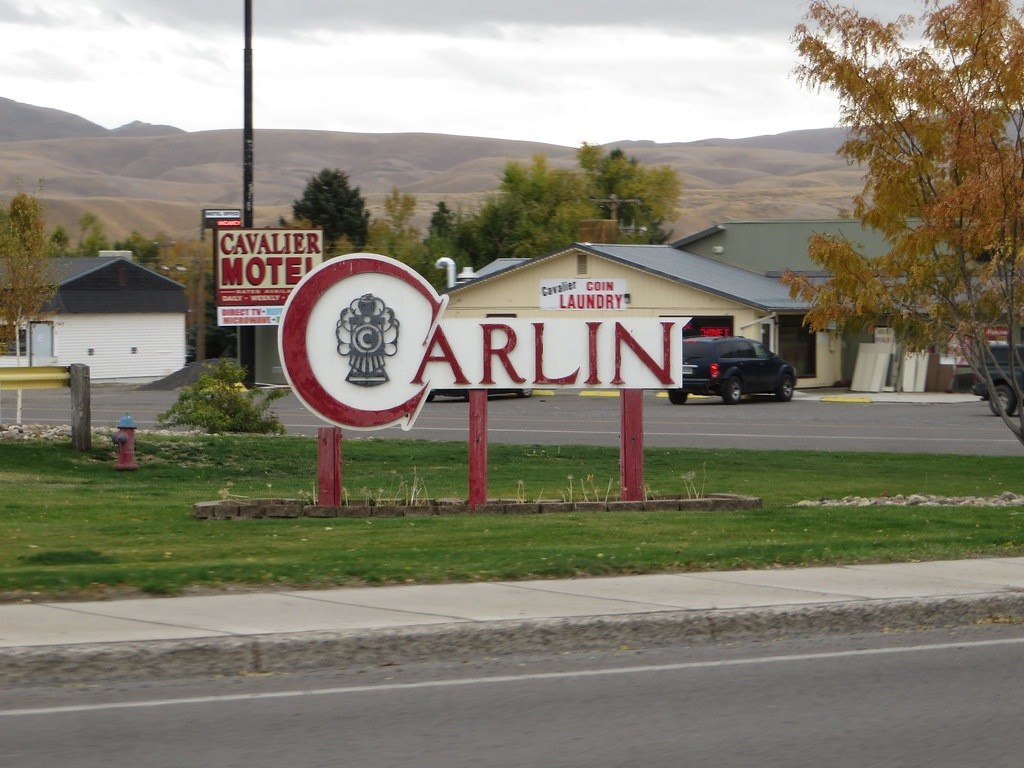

[973,345,1024,416]
[668,334,798,403]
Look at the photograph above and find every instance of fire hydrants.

[112,412,141,473]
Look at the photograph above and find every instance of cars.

[185,325,230,361]
[428,386,535,400]
[10,329,26,352]
